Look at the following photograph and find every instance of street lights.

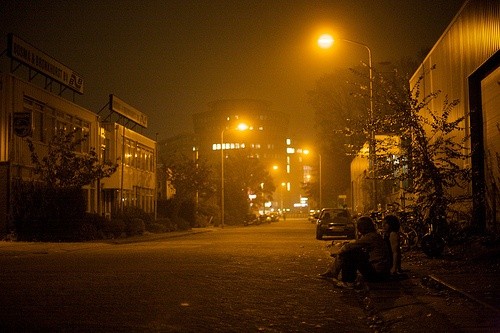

[303,150,322,214]
[318,34,377,211]
[220,122,247,227]
[262,165,278,215]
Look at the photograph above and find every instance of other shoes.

[318,270,337,281]
[335,281,353,289]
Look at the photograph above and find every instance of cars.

[241,211,281,227]
[314,208,356,240]
[309,214,319,224]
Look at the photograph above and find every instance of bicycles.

[369,209,424,252]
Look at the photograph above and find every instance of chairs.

[323,212,330,218]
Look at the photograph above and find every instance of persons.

[318,215,388,290]
[378,214,401,275]
